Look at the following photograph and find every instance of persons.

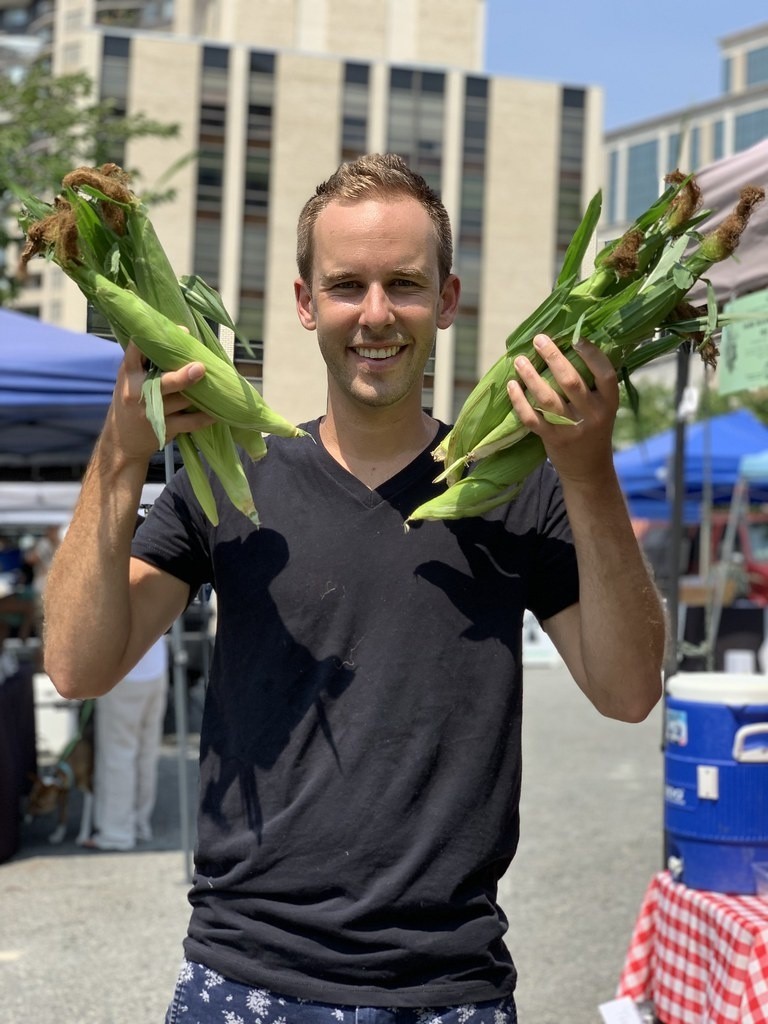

[82,634,168,850]
[44,154,666,1024]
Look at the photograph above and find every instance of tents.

[613,407,768,520]
[0,306,191,880]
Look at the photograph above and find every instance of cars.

[153,585,210,691]
[690,507,767,612]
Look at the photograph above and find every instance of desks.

[615,870,768,1024]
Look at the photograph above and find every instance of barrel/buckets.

[663,669,768,896]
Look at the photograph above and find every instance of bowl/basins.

[749,862,768,905]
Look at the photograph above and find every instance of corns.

[20,164,312,530]
[403,172,766,530]
